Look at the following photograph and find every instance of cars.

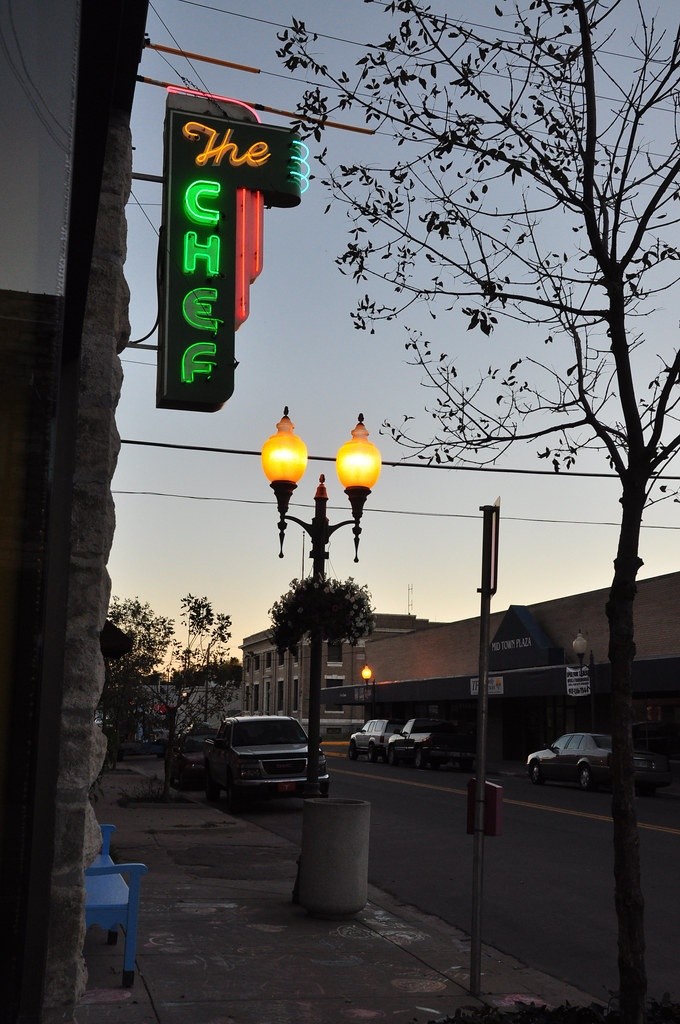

[169,728,218,790]
[143,729,170,758]
[524,733,671,795]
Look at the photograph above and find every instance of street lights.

[571,631,589,733]
[259,405,385,916]
[358,661,381,722]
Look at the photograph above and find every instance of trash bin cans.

[298,797,372,922]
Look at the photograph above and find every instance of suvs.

[205,714,330,804]
[347,716,407,763]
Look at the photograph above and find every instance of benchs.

[84,824,149,990]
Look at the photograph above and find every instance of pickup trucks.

[386,718,478,773]
